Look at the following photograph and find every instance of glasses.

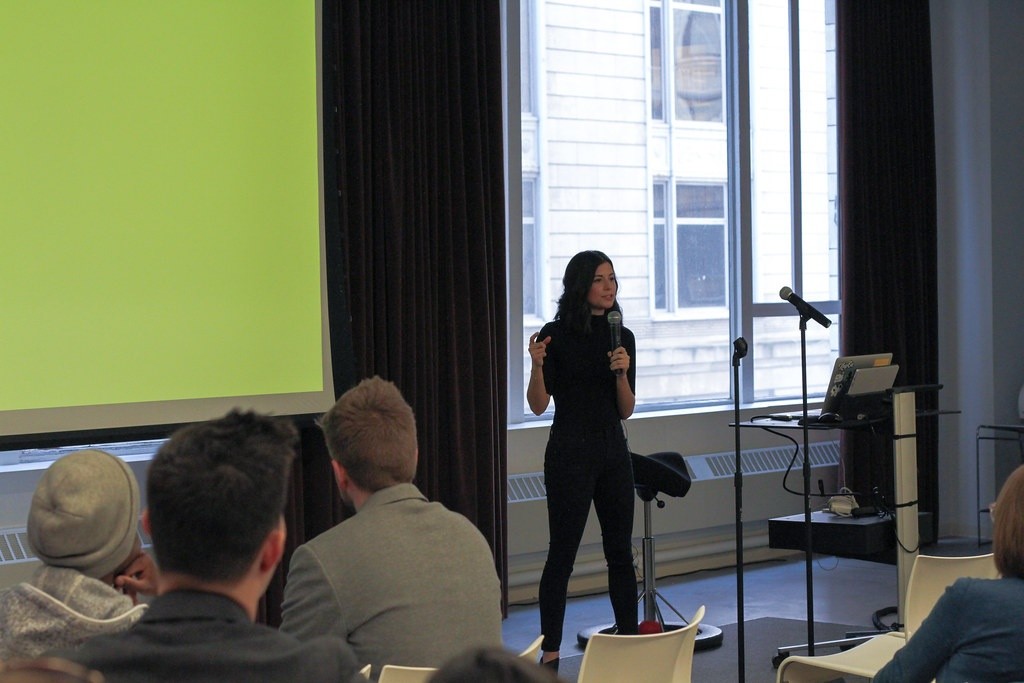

[988,501,994,521]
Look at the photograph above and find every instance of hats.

[26,448,141,579]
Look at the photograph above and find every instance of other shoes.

[539,655,558,676]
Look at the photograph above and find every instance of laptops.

[770,352,894,420]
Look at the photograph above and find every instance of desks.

[728,407,962,669]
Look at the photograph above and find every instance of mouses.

[817,412,842,423]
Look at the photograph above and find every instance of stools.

[576,450,722,653]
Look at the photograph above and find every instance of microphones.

[780,286,831,328]
[607,310,623,377]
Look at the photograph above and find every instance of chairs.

[377,635,545,683]
[775,552,1002,683]
[578,605,706,683]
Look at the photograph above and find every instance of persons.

[422,645,567,682]
[876,468,1021,681]
[69,407,360,683]
[526,251,637,672]
[71,447,155,647]
[284,375,504,683]
